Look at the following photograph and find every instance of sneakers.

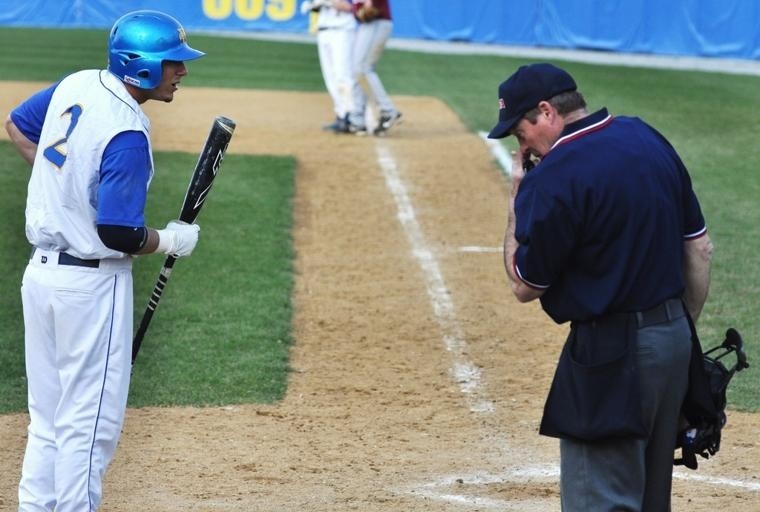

[375,109,402,136]
[324,113,367,136]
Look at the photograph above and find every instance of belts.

[640,296,686,328]
[29,246,98,267]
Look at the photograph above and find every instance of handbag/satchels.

[539,311,649,443]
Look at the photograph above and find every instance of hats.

[486,62,577,138]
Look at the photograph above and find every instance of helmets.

[108,10,205,91]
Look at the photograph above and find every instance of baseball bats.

[132,116,237,366]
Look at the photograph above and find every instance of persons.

[300,0,406,137]
[4,10,201,512]
[487,64,714,512]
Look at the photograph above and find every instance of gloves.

[151,220,200,257]
